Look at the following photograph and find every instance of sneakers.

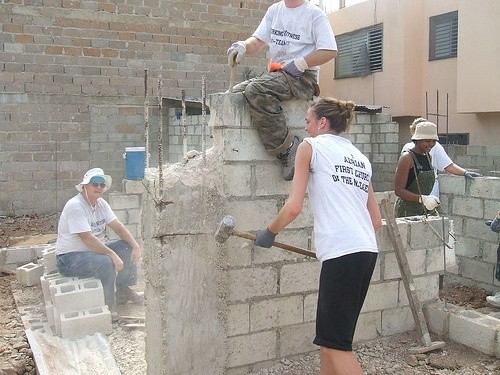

[276,135,304,182]
[486,292,500,308]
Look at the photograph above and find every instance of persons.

[228,0,338,181]
[400,117,482,197]
[394,122,440,217]
[54,167,144,321]
[253,97,380,374]
[486,210,500,309]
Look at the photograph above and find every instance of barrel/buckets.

[122,147,145,180]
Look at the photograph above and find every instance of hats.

[75,168,112,193]
[412,122,439,141]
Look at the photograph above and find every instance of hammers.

[213,215,316,259]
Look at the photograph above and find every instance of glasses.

[93,183,106,188]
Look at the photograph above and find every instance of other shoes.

[116,285,144,304]
[108,304,119,321]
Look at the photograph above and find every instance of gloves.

[419,195,441,211]
[254,227,278,249]
[465,170,483,178]
[228,41,247,67]
[279,56,309,76]
